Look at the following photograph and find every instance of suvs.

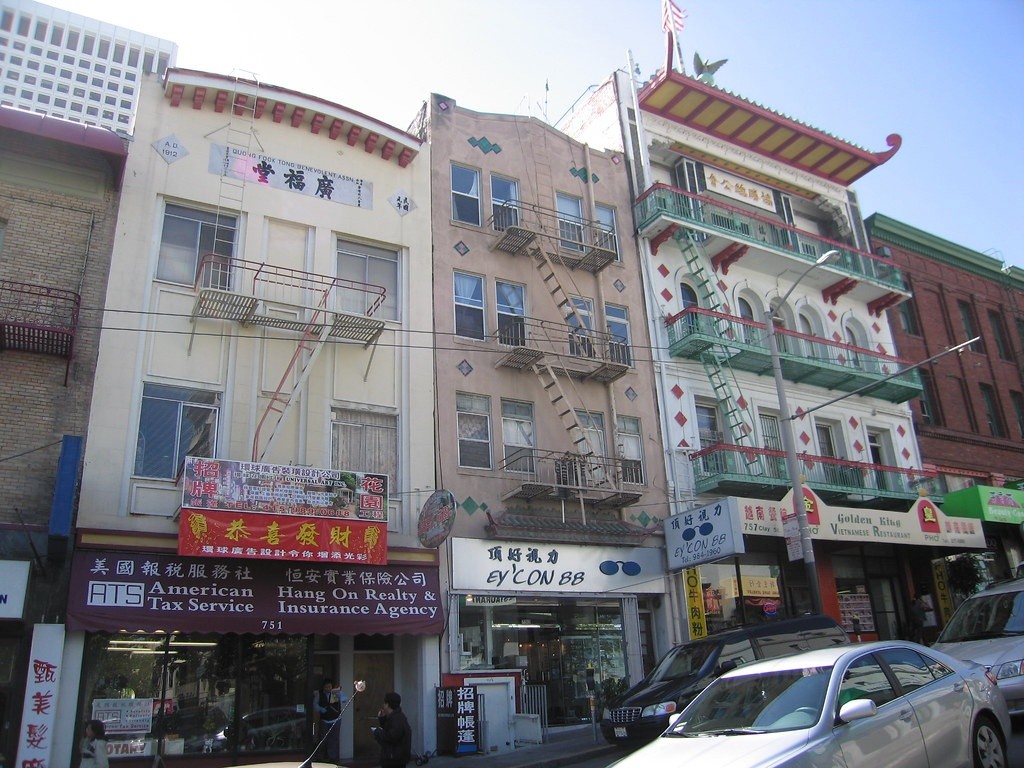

[599,612,853,744]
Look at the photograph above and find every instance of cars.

[203,706,307,755]
[928,578,1024,718]
[603,639,1010,768]
[102,706,149,740]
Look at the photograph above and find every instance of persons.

[909,599,931,647]
[313,679,348,764]
[370,691,412,768]
[79,719,110,768]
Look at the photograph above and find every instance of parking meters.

[852,619,861,643]
[585,668,597,741]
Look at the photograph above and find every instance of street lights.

[763,249,842,614]
